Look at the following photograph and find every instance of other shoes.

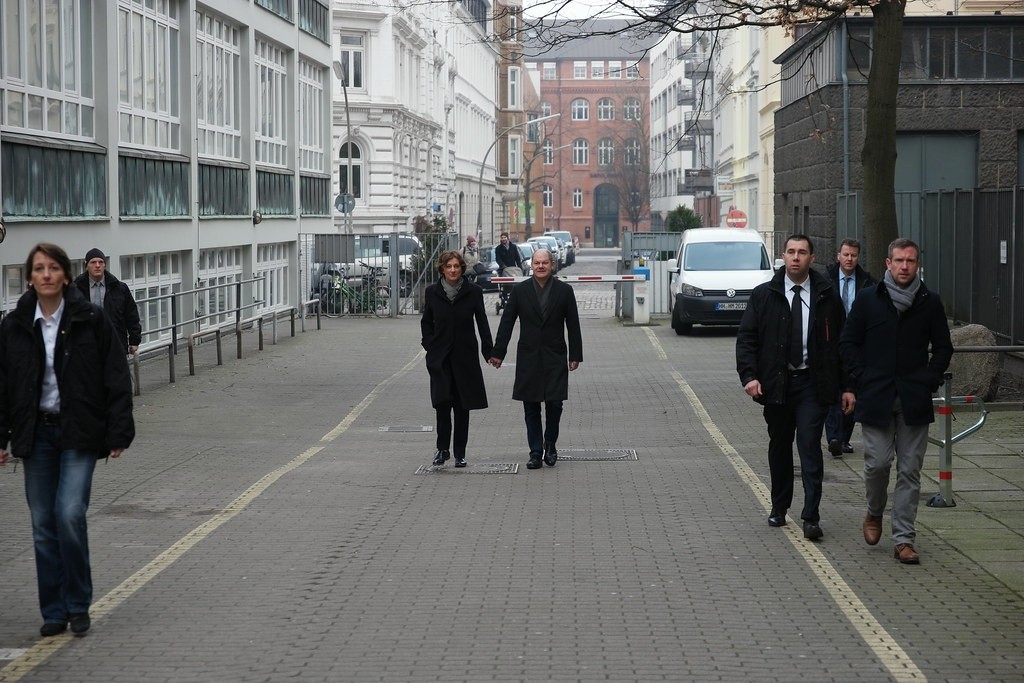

[843,441,853,453]
[70,613,90,633]
[40,621,67,636]
[828,439,842,456]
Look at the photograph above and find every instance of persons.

[420,251,494,467]
[0,244,135,636]
[458,235,480,273]
[838,239,954,563]
[490,248,583,469]
[495,232,523,301]
[736,235,856,537]
[821,238,873,456]
[74,247,142,356]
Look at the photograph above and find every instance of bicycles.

[319,261,391,318]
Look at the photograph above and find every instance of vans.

[666,227,785,336]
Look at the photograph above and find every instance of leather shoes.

[768,508,785,526]
[894,543,919,563]
[803,522,823,538]
[527,455,542,469]
[433,449,450,465]
[544,442,557,466]
[455,457,466,467]
[863,513,882,545]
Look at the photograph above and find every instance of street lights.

[332,61,354,234]
[516,143,571,237]
[478,113,561,247]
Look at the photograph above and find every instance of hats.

[467,235,476,244]
[85,248,106,267]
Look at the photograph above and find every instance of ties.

[842,277,851,319]
[95,282,101,306]
[789,285,803,370]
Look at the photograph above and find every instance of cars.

[477,231,576,289]
[312,235,425,299]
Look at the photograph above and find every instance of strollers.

[495,267,523,315]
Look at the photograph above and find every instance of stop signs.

[727,210,747,228]
